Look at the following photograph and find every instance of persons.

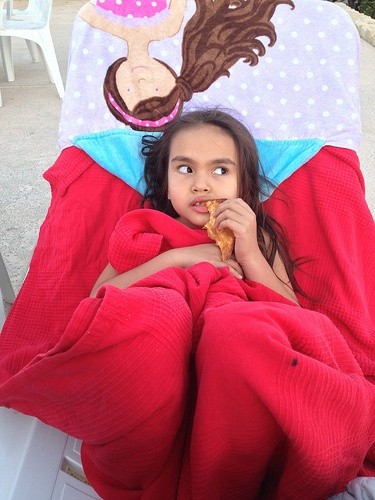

[90,103,322,305]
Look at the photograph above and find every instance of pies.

[201,202,234,262]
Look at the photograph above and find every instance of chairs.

[0,0,65,98]
